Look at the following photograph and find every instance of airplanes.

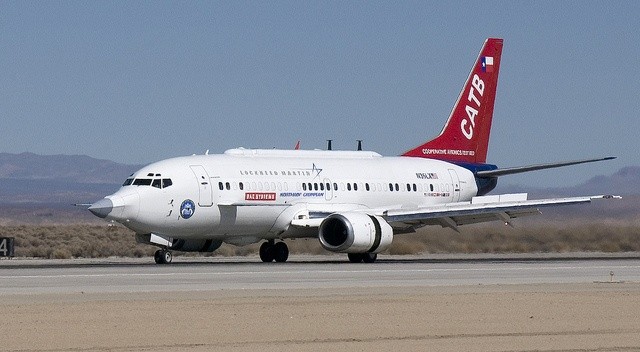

[71,38,623,263]
